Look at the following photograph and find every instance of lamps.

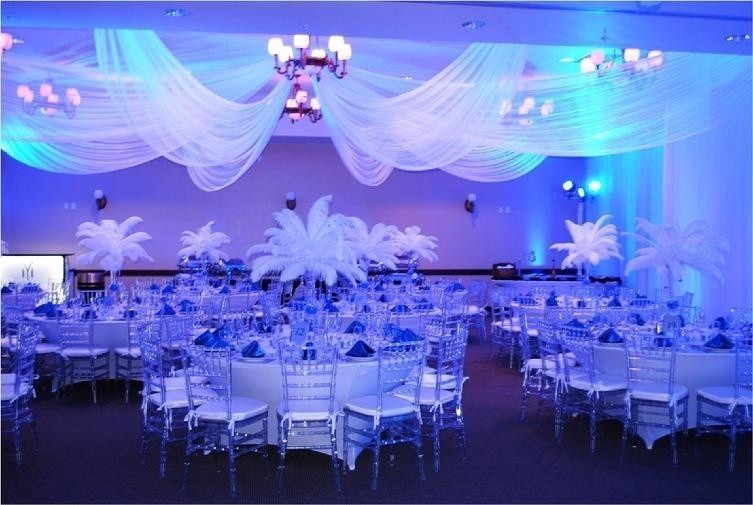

[93,189,107,211]
[561,180,587,203]
[464,193,476,214]
[286,191,296,210]
[0,33,80,119]
[268,35,351,124]
[496,28,663,126]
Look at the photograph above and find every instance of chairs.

[1,266,752,370]
[554,327,628,454]
[343,336,428,492]
[136,321,209,455]
[695,338,752,475]
[518,311,564,422]
[136,328,221,485]
[276,343,342,494]
[59,307,111,404]
[2,317,62,401]
[178,343,269,496]
[388,329,467,473]
[1,317,40,468]
[533,319,598,429]
[622,332,689,465]
[406,324,470,437]
[114,303,164,406]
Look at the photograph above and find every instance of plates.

[32,294,196,321]
[315,308,440,317]
[562,314,753,353]
[191,322,417,364]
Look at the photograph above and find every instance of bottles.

[302,330,319,364]
